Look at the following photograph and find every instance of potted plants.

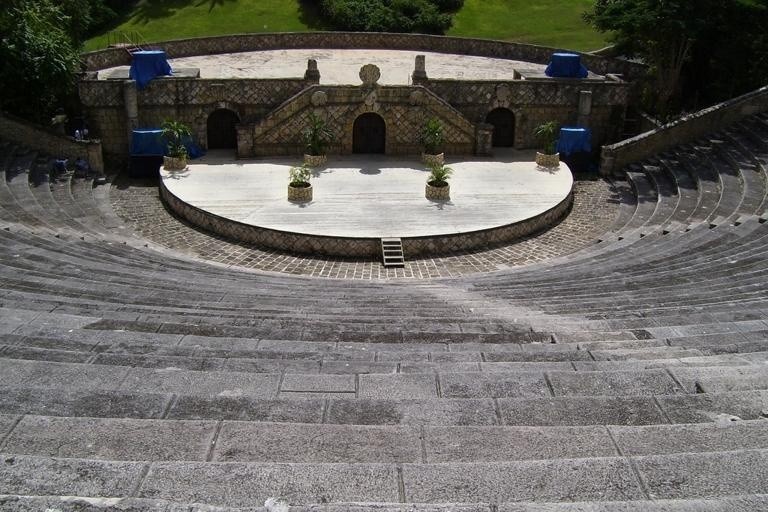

[534,121,562,167]
[426,162,453,198]
[289,163,312,202]
[421,115,445,166]
[302,115,331,167]
[162,122,192,172]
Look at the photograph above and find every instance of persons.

[73,125,89,139]
